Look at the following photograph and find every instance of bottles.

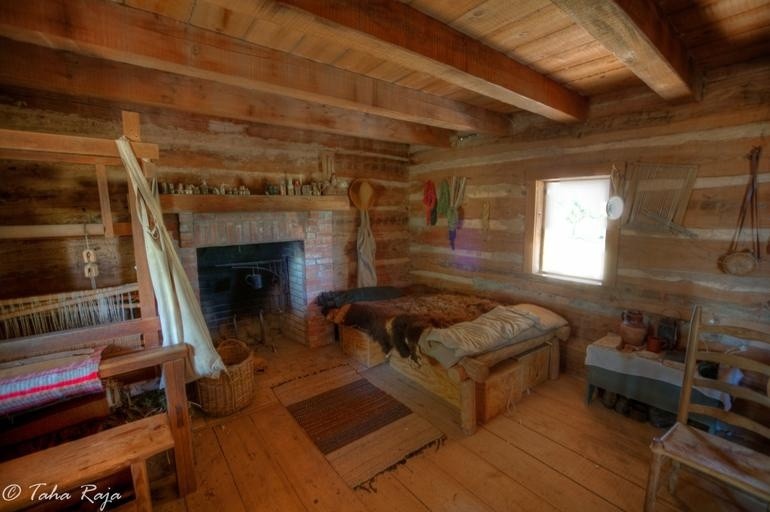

[280,179,301,196]
[620,308,648,347]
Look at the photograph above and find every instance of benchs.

[1,413,175,511]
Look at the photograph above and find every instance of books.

[594,334,624,350]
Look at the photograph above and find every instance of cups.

[647,336,668,352]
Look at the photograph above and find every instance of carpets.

[269,361,449,492]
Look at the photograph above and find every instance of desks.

[583,331,747,441]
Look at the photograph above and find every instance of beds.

[315,282,574,438]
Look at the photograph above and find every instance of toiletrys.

[287,178,294,195]
[295,181,303,196]
[280,181,286,196]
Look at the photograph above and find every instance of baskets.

[196,336,256,419]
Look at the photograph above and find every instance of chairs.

[644,301,770,510]
